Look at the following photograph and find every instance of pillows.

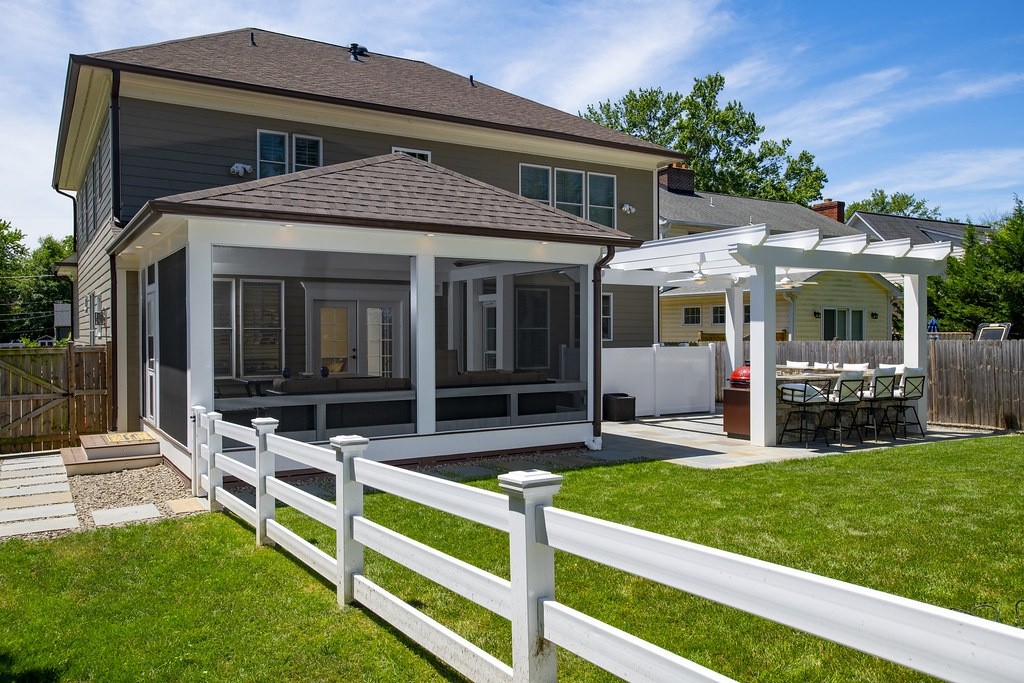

[776,384,824,395]
[877,363,906,385]
[843,362,869,370]
[785,361,809,368]
[813,362,839,368]
[868,367,896,392]
[833,371,864,397]
[898,367,924,395]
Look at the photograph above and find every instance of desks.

[233,378,275,396]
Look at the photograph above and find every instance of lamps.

[230,163,253,176]
[694,261,707,284]
[622,204,636,214]
[871,312,878,319]
[781,267,793,282]
[814,311,821,318]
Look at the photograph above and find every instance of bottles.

[321,367,329,378]
[283,368,290,379]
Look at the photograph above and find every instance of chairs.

[813,379,865,445]
[877,375,926,440]
[846,376,897,442]
[779,378,832,447]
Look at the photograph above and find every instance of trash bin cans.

[723,366,751,440]
[604,392,636,422]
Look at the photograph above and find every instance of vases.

[283,367,291,378]
[320,367,330,378]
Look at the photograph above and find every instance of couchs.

[266,369,558,431]
